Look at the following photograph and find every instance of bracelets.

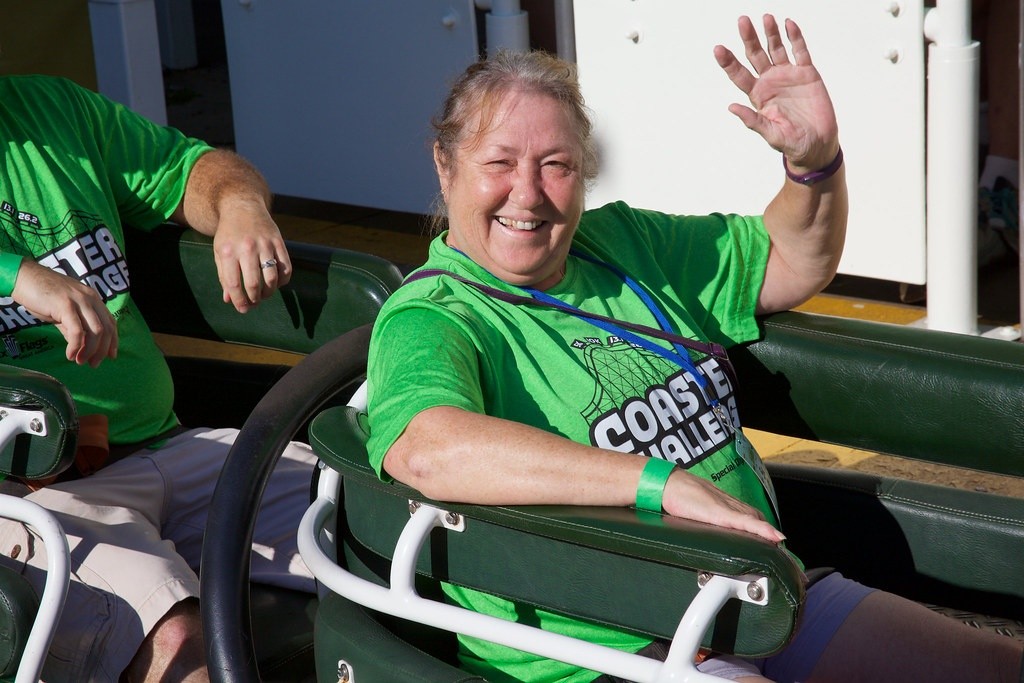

[783,144,843,186]
[635,456,678,513]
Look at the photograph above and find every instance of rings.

[260,259,277,268]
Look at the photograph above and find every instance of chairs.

[0,225,1024,683]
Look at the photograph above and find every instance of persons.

[366,12,1024,683]
[0,72,330,683]
